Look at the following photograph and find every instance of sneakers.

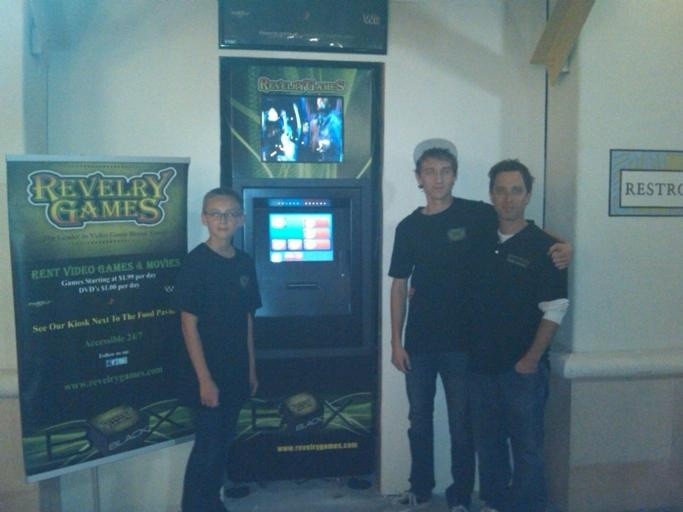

[389,491,500,512]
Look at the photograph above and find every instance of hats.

[413,138,458,167]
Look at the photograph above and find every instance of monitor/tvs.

[268,213,335,263]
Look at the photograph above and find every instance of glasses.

[204,210,242,221]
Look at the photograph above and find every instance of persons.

[408,158,570,511]
[386,137,573,511]
[175,187,263,511]
